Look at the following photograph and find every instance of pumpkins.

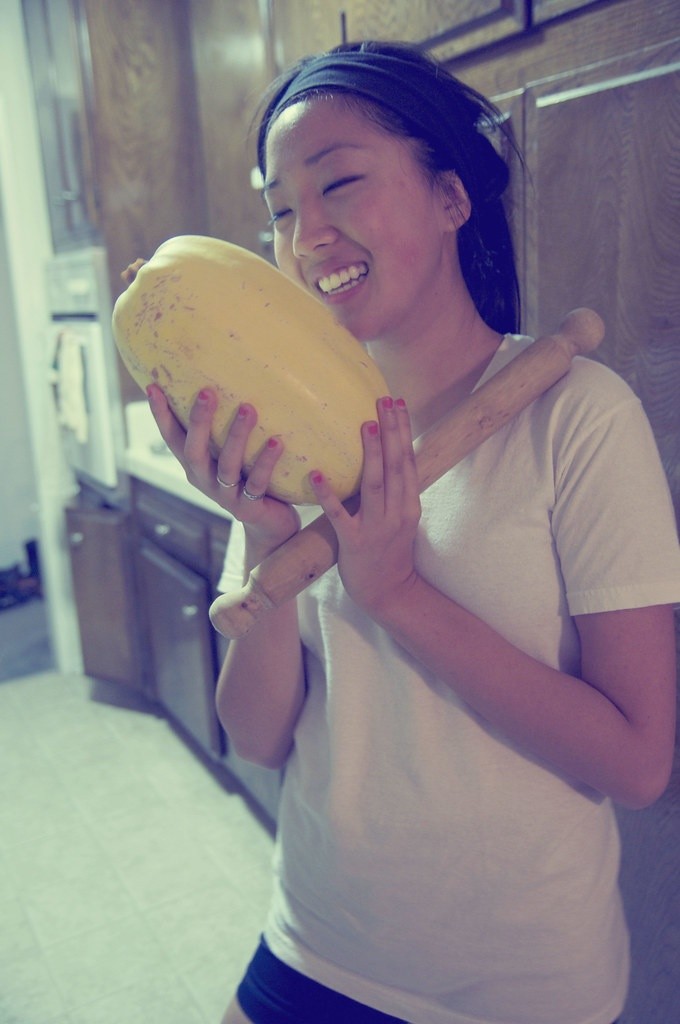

[109,236,393,505]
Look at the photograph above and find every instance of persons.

[147,43,680,1024]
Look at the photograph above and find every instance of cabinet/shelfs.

[22,2,680,1024]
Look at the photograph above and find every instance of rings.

[243,486,265,501]
[217,475,241,488]
